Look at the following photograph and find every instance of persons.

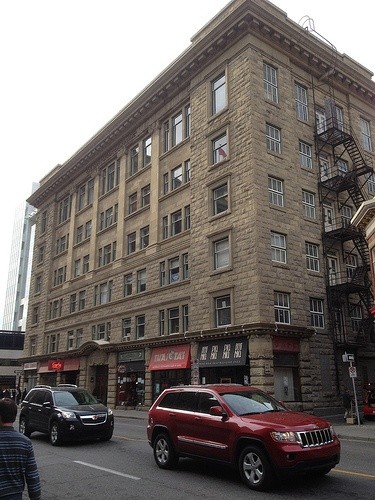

[342,384,355,424]
[0,398,41,500]
[0,387,29,406]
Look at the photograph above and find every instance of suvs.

[18,383,115,447]
[146,381,341,491]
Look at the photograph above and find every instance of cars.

[361,381,375,418]
